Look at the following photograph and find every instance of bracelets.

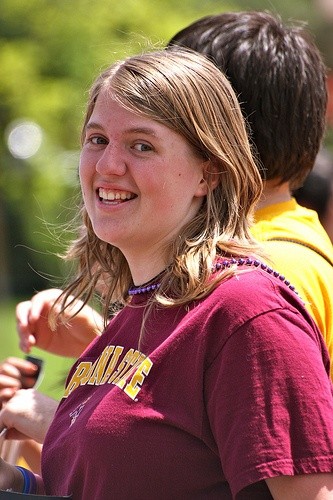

[14,465,38,495]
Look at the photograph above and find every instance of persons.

[1,230,138,472]
[15,7,333,384]
[1,47,333,500]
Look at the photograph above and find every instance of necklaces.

[127,258,300,297]
